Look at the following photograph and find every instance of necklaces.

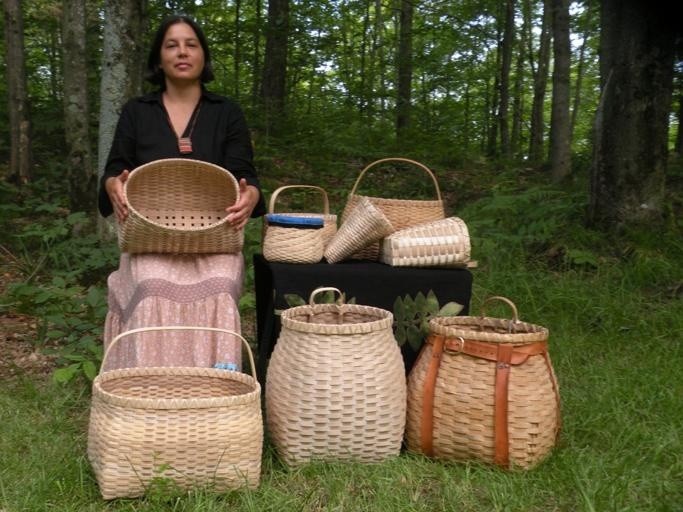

[167,102,201,155]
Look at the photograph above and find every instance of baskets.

[86,287,561,501]
[114,157,472,269]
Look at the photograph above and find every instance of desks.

[252,251,474,389]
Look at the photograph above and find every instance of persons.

[96,13,267,374]
